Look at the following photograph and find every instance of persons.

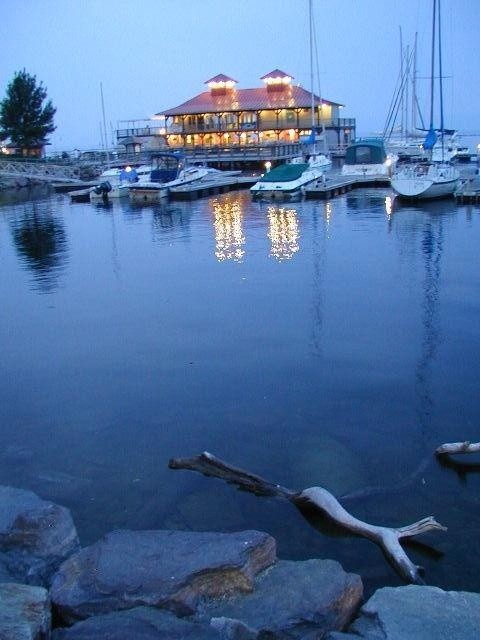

[61,148,80,165]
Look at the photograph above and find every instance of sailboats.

[50,80,118,160]
[391,0,461,202]
[381,26,469,162]
[289,1,334,172]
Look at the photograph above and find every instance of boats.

[51,151,244,202]
[249,162,326,199]
[340,138,400,177]
